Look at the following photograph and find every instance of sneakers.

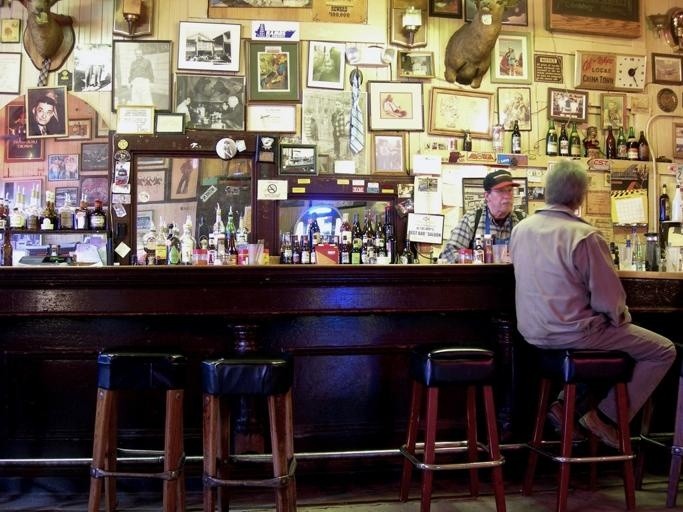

[548,401,584,442]
[579,408,620,448]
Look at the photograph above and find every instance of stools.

[519,349,637,512]
[635,354,683,506]
[201,358,301,511]
[88,352,187,512]
[399,345,507,512]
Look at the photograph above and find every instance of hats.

[484,170,520,190]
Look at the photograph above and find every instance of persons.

[9,46,628,195]
[509,160,677,449]
[443,170,528,264]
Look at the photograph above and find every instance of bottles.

[0,185,418,265]
[448,117,682,271]
